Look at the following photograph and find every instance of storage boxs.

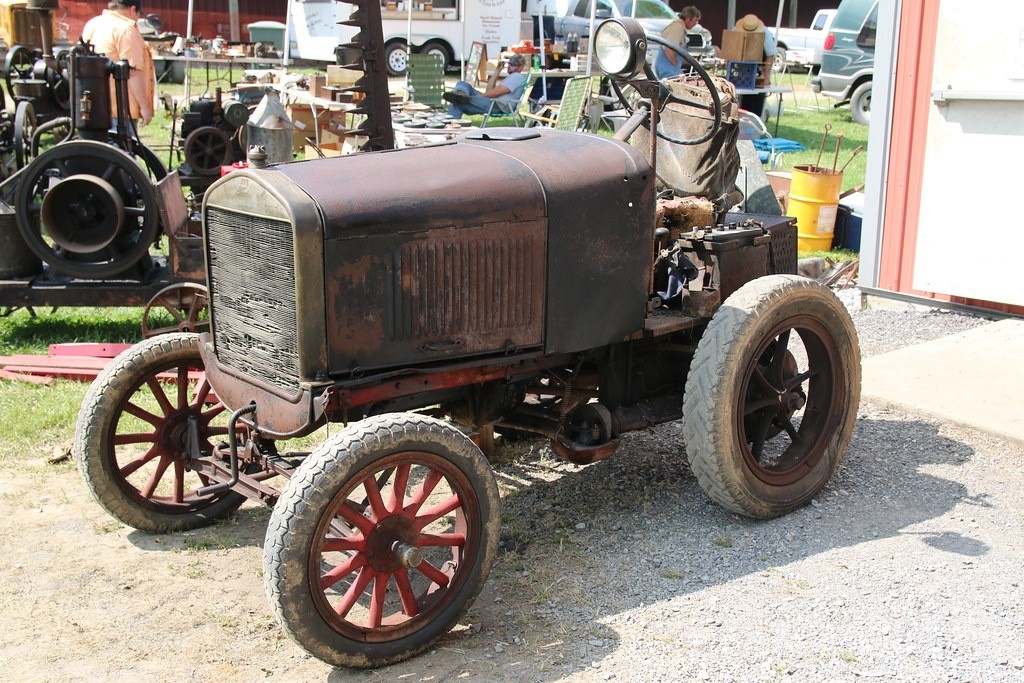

[231,45,247,54]
[285,64,368,160]
[832,192,865,253]
[726,61,758,90]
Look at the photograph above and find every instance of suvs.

[809,1,882,125]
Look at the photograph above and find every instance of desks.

[392,122,478,141]
[735,85,792,138]
[152,55,294,113]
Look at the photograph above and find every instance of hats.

[508,55,525,66]
[735,14,765,37]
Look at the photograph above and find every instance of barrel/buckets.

[786,165,843,252]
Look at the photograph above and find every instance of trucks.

[285,1,550,82]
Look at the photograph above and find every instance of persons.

[732,13,777,114]
[655,6,700,79]
[443,54,526,120]
[81,0,154,137]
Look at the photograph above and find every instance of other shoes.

[442,92,470,105]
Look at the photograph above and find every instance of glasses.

[507,63,520,67]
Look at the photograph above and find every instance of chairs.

[737,108,783,172]
[479,71,532,128]
[598,76,647,132]
[406,53,448,110]
[554,76,597,135]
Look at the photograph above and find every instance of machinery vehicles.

[74,15,864,670]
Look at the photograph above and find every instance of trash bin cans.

[246,20,286,70]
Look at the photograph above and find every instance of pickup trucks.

[766,9,837,74]
[551,0,726,72]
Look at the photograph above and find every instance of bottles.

[738,117,754,140]
[572,33,578,52]
[566,33,572,52]
[212,35,224,51]
[535,54,539,72]
[554,35,564,45]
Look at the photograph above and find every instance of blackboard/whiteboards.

[464,41,489,82]
